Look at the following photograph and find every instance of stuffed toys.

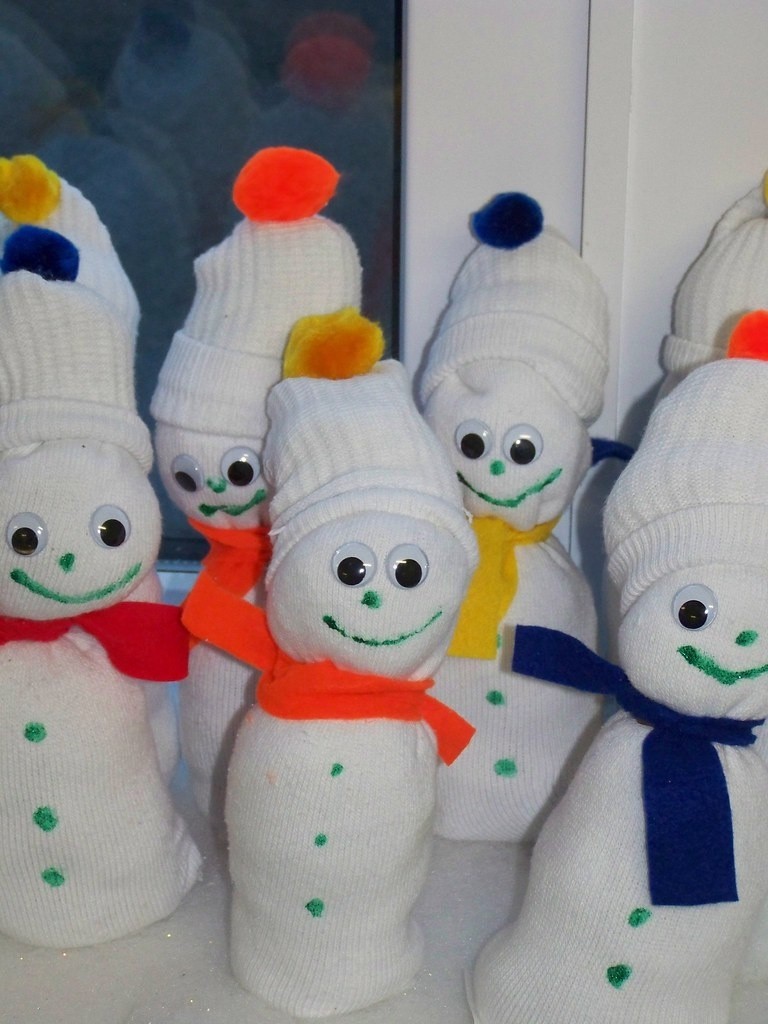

[0,148,768,1024]
